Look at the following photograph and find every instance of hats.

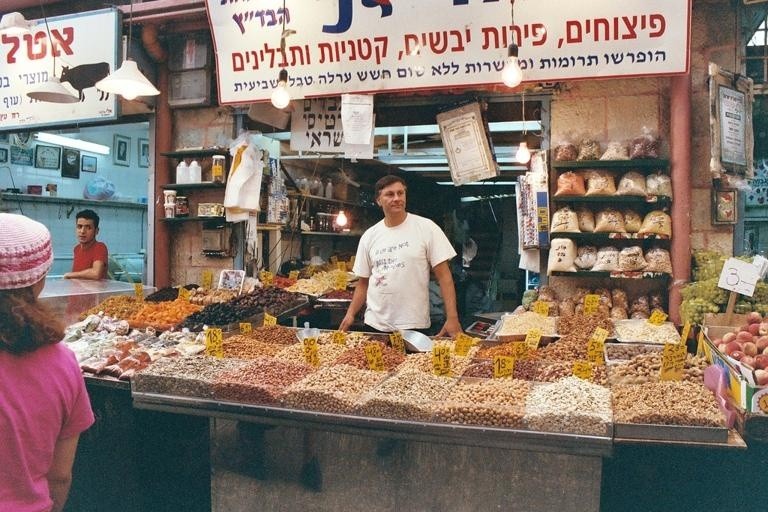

[0,212,54,291]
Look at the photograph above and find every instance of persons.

[64,208,109,320]
[336,173,465,338]
[0,209,100,512]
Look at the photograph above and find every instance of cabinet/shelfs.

[551,159,672,280]
[158,148,231,223]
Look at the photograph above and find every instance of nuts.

[79,136,729,437]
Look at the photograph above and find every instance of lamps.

[0,1,162,104]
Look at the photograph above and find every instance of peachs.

[711,310,767,386]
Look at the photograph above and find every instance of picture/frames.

[0,135,97,179]
[112,134,149,167]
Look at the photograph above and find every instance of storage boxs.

[694,312,768,415]
